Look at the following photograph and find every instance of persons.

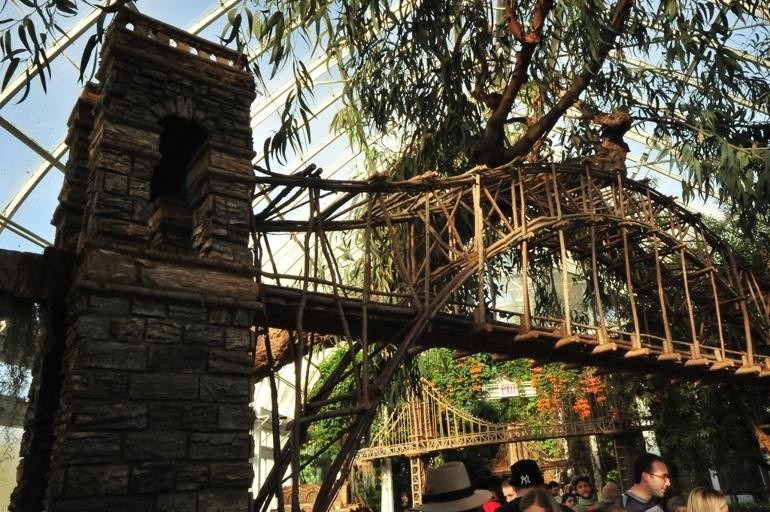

[477,474,507,511]
[665,492,688,512]
[686,485,730,511]
[501,476,522,503]
[618,451,672,511]
[492,457,576,512]
[546,480,563,504]
[568,473,601,511]
[559,492,578,509]
[517,486,561,512]
[581,478,627,511]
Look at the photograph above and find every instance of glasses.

[649,474,668,482]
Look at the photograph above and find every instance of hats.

[408,461,492,511]
[508,459,545,487]
[600,481,622,504]
